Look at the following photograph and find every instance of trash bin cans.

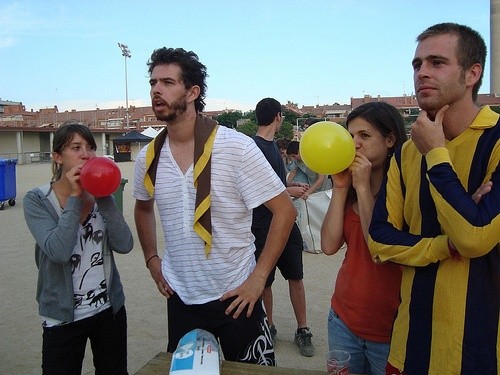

[1,158,17,209]
[111,178,129,215]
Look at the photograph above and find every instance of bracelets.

[146,254,159,268]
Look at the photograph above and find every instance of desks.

[134,352,360,375]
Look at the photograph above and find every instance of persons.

[133,47,298,367]
[23,123,135,375]
[245,98,316,356]
[273,138,338,204]
[367,24,500,375]
[320,101,407,375]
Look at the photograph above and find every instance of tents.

[112,126,166,162]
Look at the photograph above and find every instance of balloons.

[80,157,121,198]
[299,121,356,175]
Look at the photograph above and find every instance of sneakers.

[267,323,277,340]
[293,327,316,358]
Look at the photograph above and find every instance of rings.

[410,133,414,137]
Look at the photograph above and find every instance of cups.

[325,350,352,375]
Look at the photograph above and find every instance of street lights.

[118,43,132,130]
